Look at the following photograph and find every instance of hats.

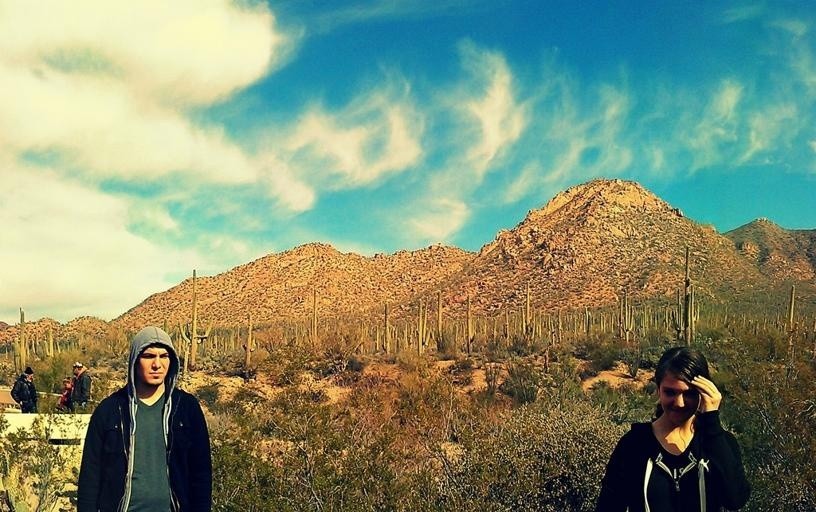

[23,367,33,374]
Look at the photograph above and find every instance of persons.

[70,361,91,406]
[594,347,752,511]
[76,325,212,511]
[53,376,73,413]
[10,367,37,412]
